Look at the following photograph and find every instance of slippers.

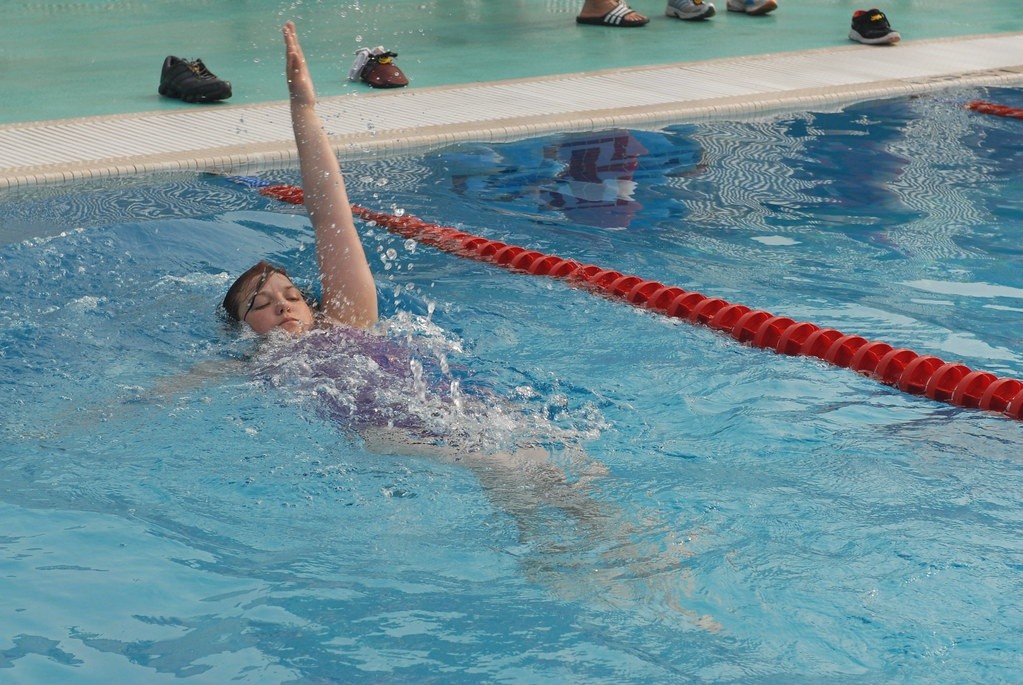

[576,2,650,27]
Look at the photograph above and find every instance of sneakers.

[848,9,901,44]
[665,0,716,20]
[727,0,778,14]
[158,55,233,103]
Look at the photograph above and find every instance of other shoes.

[360,51,409,87]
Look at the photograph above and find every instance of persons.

[5,20,733,636]
[576,0,650,27]
